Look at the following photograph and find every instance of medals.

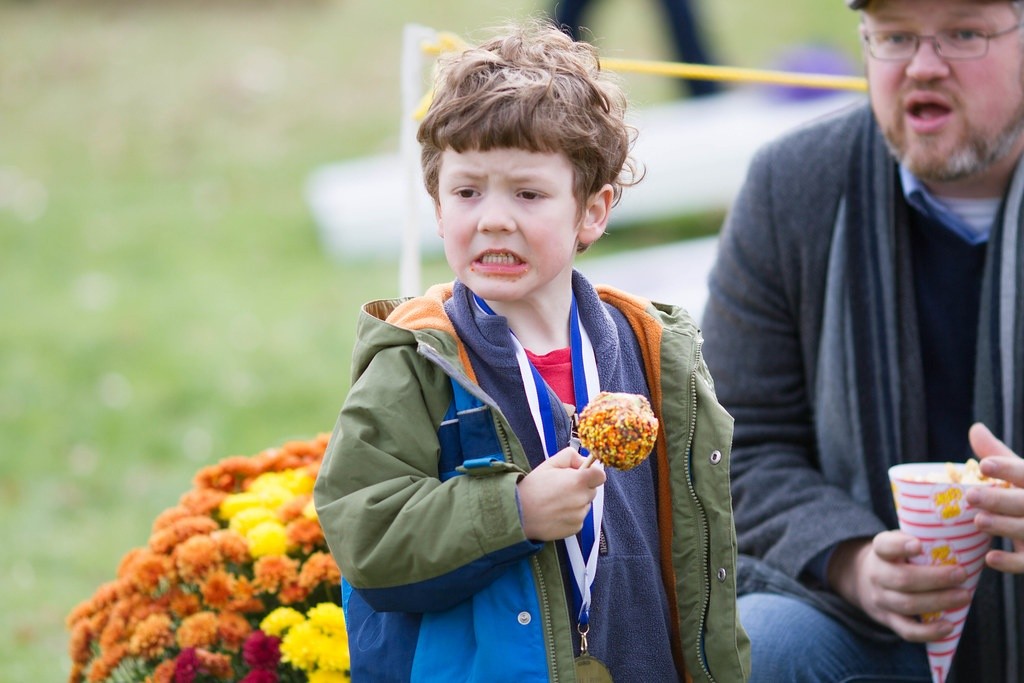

[573,655,613,683]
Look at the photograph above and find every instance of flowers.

[64,430,353,683]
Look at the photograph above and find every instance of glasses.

[861,21,1021,61]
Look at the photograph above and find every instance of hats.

[849,1,874,12]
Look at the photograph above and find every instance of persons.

[314,33,752,683]
[698,0,1024,683]
[548,1,723,101]
